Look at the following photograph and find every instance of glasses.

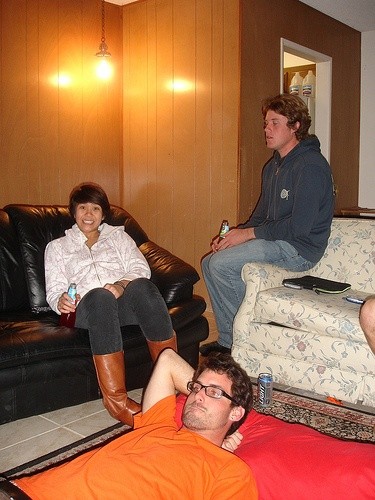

[187,381,238,407]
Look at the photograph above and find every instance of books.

[283,275,352,294]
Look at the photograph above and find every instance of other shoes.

[199,340,232,355]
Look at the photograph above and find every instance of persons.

[359,296,375,355]
[197,93,336,360]
[0,348,261,500]
[43,181,179,428]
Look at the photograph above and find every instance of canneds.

[257,373,273,407]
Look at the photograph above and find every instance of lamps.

[94,0,115,82]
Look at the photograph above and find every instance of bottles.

[302,70,315,97]
[60,284,77,328]
[217,220,229,244]
[289,72,304,96]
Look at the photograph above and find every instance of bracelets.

[113,281,126,289]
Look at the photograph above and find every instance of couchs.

[0,204,211,426]
[231,217,375,408]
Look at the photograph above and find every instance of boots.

[147,330,178,364]
[92,350,141,428]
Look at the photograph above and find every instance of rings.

[57,305,62,308]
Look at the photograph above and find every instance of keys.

[284,281,303,289]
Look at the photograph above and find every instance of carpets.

[0,381,375,500]
